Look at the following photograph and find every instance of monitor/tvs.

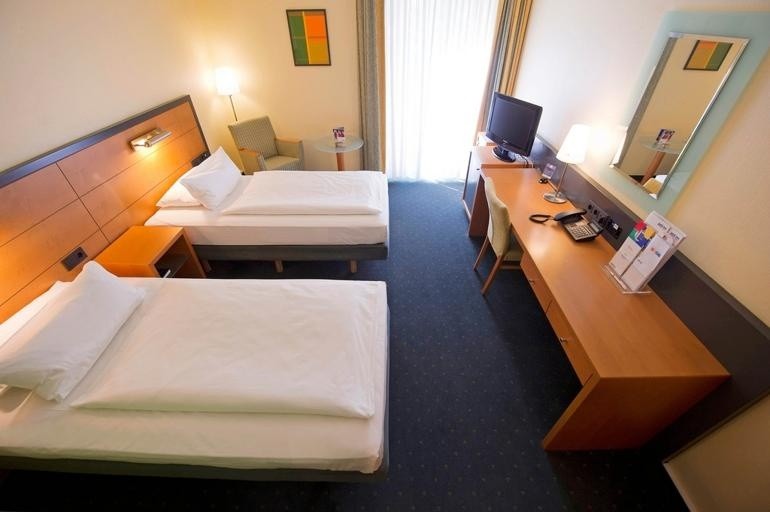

[485,92,542,162]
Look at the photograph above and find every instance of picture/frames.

[285,8,331,67]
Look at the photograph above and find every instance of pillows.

[1,259,143,400]
[1,280,62,349]
[157,177,201,207]
[181,146,243,211]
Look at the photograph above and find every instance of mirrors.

[609,30,751,201]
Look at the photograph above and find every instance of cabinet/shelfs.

[461,146,527,222]
[93,224,206,279]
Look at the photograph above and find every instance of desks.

[313,133,365,172]
[467,167,731,451]
[639,134,685,186]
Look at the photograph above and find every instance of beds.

[144,170,387,275]
[0,281,390,481]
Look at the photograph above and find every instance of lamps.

[542,123,591,204]
[132,130,171,155]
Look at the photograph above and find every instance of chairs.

[473,176,524,295]
[226,114,305,173]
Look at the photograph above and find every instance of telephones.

[555,208,603,242]
